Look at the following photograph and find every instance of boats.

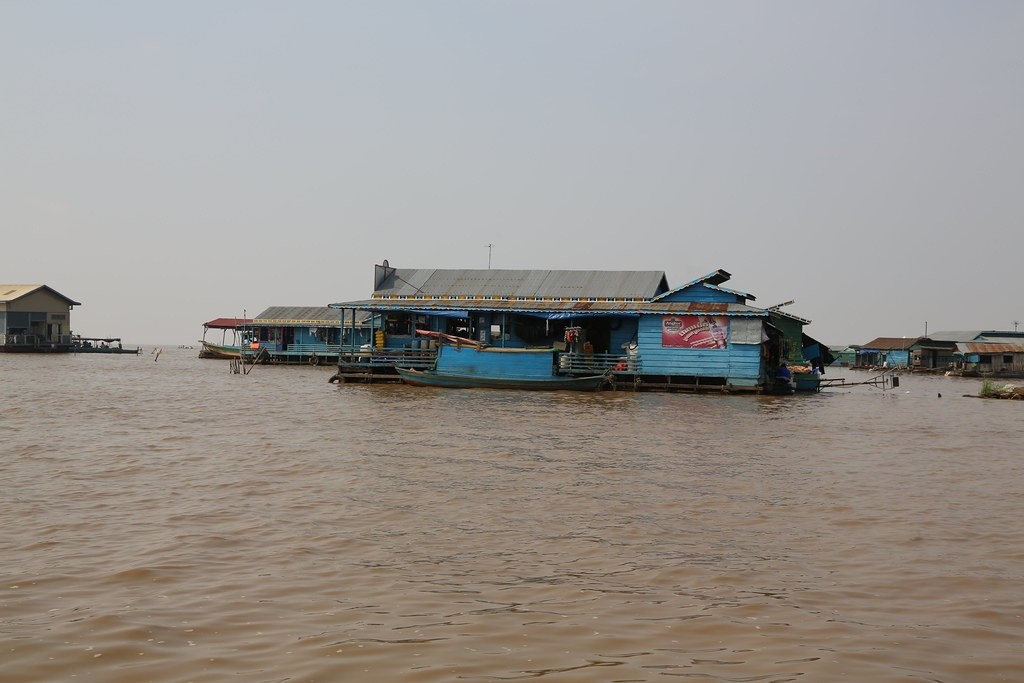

[395,344,619,394]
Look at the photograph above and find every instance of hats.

[630,336,638,342]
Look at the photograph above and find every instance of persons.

[96,342,105,348]
[359,338,379,363]
